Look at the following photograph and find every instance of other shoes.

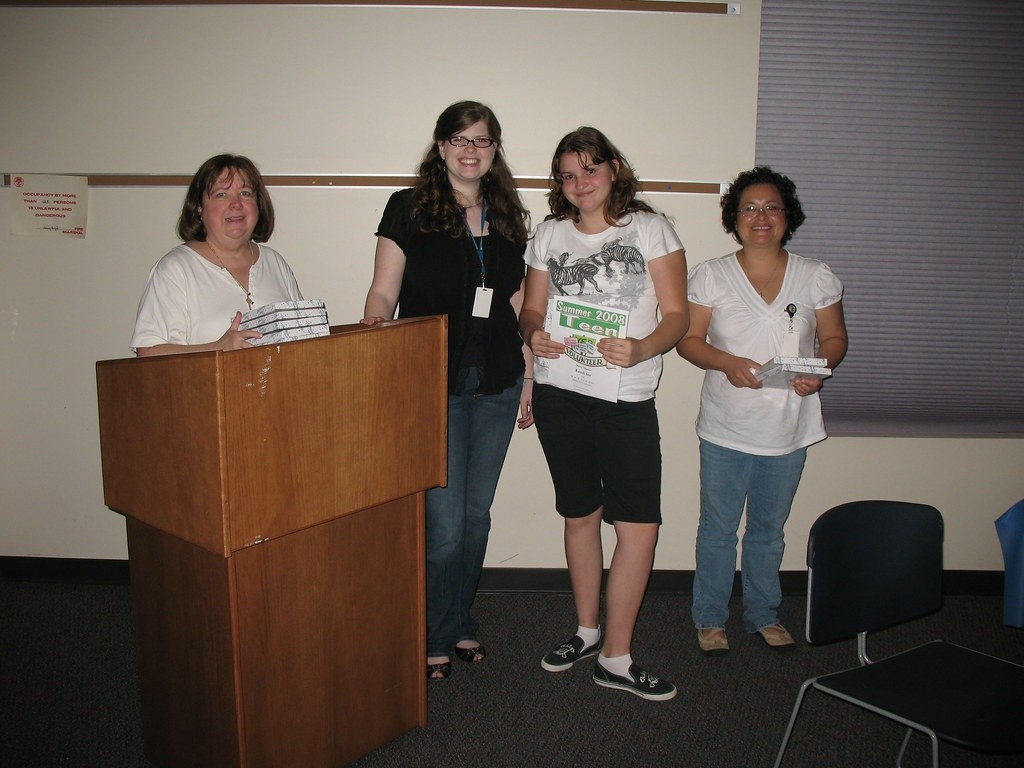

[755,624,792,647]
[697,628,730,656]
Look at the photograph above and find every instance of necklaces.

[741,251,781,298]
[206,240,256,311]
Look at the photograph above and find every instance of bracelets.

[523,377,533,380]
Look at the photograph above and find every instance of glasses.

[737,203,785,217]
[446,136,496,148]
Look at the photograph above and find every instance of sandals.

[427,661,451,681]
[455,645,486,662]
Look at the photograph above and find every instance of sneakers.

[541,629,603,672]
[593,653,677,701]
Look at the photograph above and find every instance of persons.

[359,100,535,681]
[674,165,849,653]
[518,127,692,701]
[128,152,306,361]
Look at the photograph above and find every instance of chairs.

[771,497,1024,767]
